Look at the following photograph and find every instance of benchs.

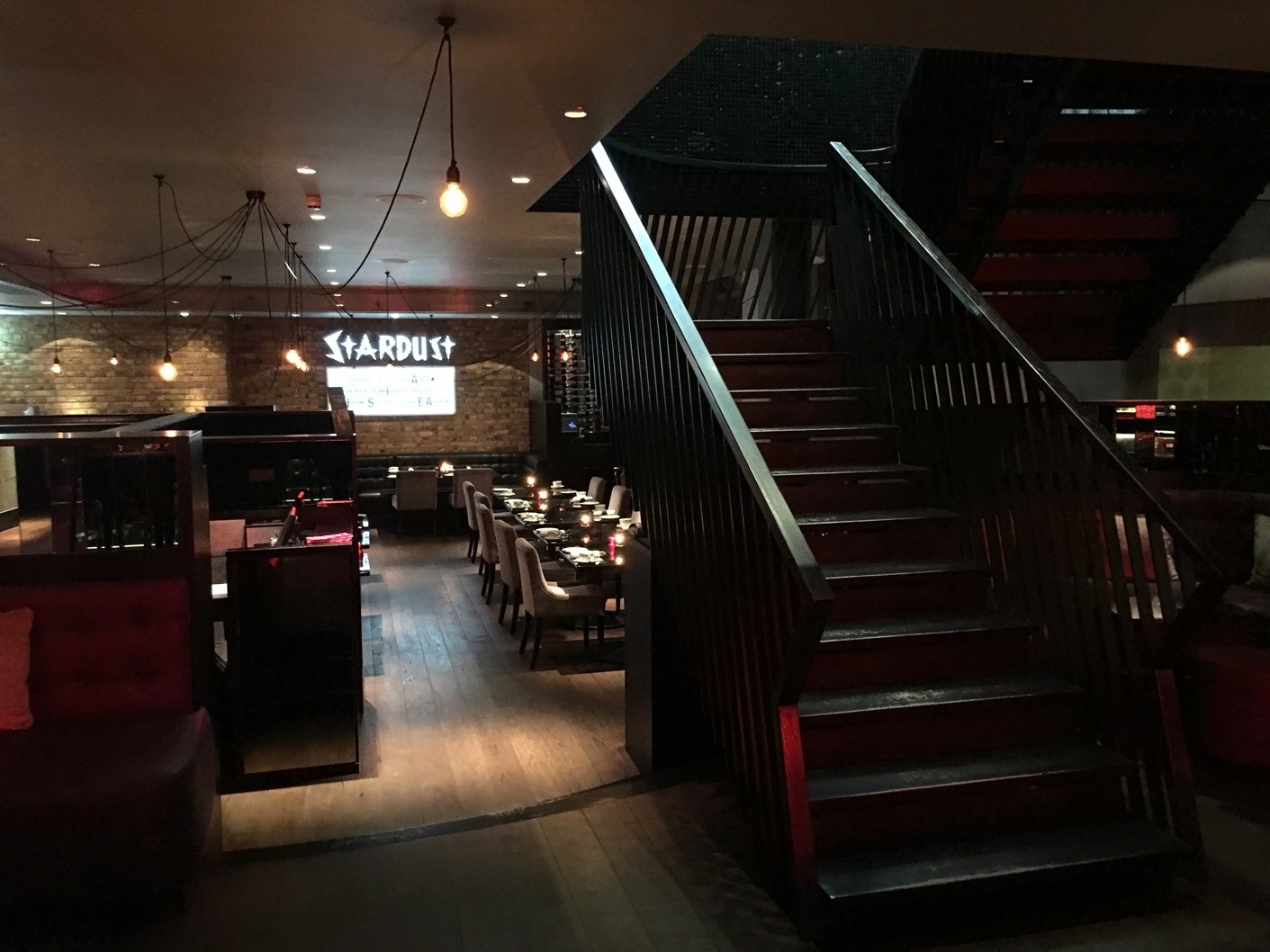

[356,450,546,529]
[0,410,362,916]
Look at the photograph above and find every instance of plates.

[500,494,515,496]
[524,519,540,522]
[573,498,592,500]
[544,537,561,539]
[602,516,620,518]
[511,506,524,508]
[551,485,564,487]
[574,559,603,562]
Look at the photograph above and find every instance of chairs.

[588,477,605,502]
[448,468,494,533]
[391,470,437,539]
[608,485,632,518]
[463,481,606,671]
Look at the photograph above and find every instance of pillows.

[0,607,33,731]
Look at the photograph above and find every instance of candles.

[527,476,625,548]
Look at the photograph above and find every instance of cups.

[595,505,605,511]
[577,492,586,498]
[548,531,560,538]
[580,552,593,560]
[607,511,614,515]
[514,501,523,507]
[503,489,512,494]
[527,513,537,520]
[553,481,561,486]
[619,519,631,526]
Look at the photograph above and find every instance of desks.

[491,485,623,656]
